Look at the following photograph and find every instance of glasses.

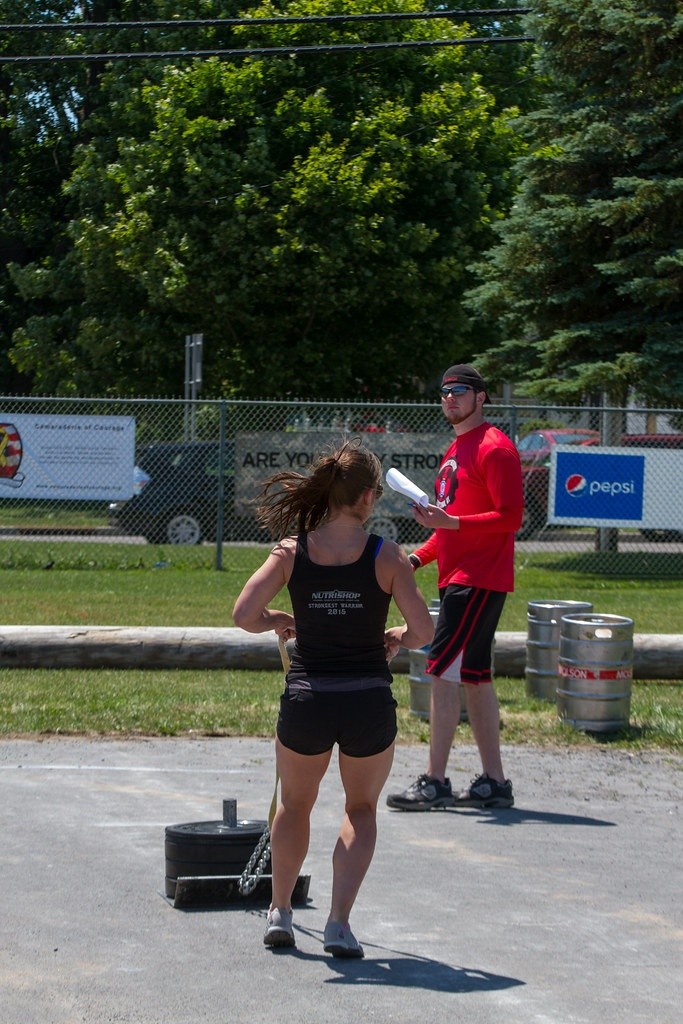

[439,385,475,398]
[360,484,383,498]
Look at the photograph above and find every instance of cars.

[109,404,682,547]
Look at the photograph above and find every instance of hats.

[441,364,492,404]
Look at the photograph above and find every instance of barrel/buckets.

[525,599,592,706]
[556,614,634,741]
[408,607,468,721]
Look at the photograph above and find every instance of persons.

[231,431,435,956]
[386,365,524,810]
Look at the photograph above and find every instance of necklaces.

[320,524,363,529]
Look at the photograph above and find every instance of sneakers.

[263,902,295,946]
[387,773,455,811]
[453,772,514,808]
[324,919,364,957]
